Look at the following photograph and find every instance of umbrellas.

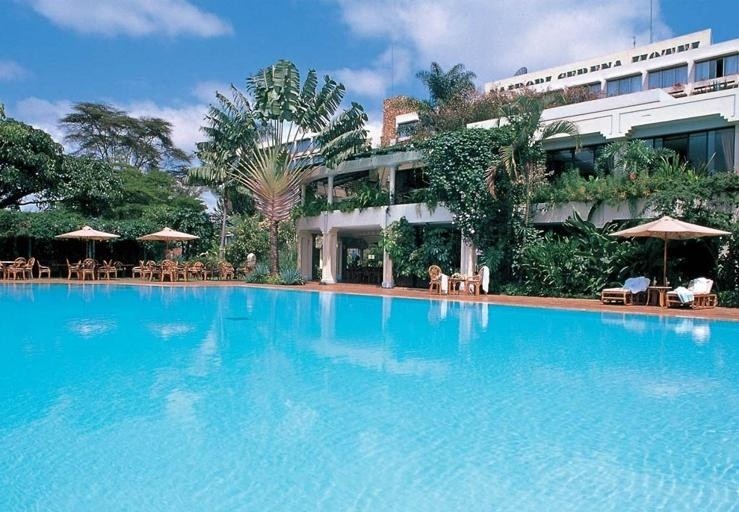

[606,209,736,288]
[54,224,122,262]
[135,225,201,262]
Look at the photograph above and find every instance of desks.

[647,286,671,307]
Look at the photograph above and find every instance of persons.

[346,250,361,269]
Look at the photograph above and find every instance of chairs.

[217,260,256,280]
[37,260,50,279]
[429,265,483,295]
[132,260,207,282]
[666,277,717,309]
[0,257,35,280]
[67,258,117,280]
[601,276,650,305]
